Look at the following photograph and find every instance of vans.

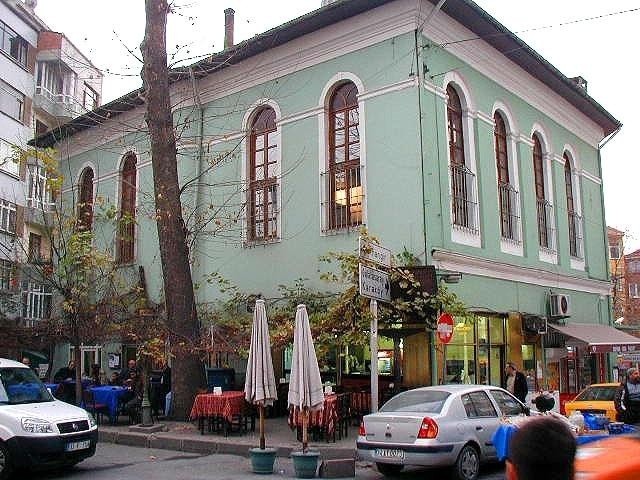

[0,358,98,480]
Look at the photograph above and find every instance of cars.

[354,385,546,480]
[565,383,626,422]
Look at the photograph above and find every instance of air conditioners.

[548,294,570,322]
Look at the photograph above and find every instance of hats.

[626,367,637,376]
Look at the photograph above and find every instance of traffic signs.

[358,236,391,304]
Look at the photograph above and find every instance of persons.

[613,368,640,425]
[22,355,171,422]
[503,361,528,404]
[503,415,577,480]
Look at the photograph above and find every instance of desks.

[44,383,141,424]
[190,390,381,441]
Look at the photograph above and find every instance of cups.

[323,392,328,400]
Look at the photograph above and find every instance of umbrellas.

[243,299,280,450]
[286,304,325,452]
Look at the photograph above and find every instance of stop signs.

[437,313,454,343]
